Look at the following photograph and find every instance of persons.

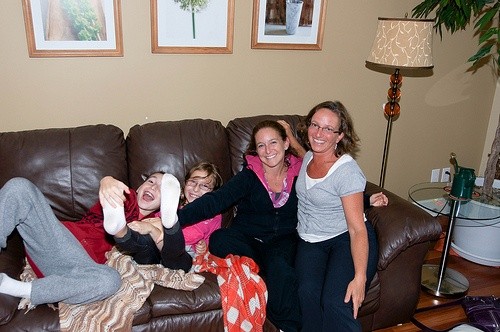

[276,100,380,332]
[141,120,388,332]
[0,171,167,306]
[102,160,224,274]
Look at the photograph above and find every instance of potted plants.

[410,0,500,259]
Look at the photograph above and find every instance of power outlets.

[441,168,450,182]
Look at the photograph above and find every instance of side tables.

[409,183,500,299]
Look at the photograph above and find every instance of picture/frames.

[149,0,235,54]
[21,0,124,58]
[251,0,328,51]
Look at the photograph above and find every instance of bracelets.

[157,239,164,244]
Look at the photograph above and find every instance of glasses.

[185,180,213,191]
[307,122,341,135]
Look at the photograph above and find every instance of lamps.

[365,12,434,189]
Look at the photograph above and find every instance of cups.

[451,166,477,201]
[285,0,303,35]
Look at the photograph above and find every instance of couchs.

[0,115,442,332]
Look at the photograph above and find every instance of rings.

[149,219,150,222]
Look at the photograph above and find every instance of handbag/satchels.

[460,294,500,332]
